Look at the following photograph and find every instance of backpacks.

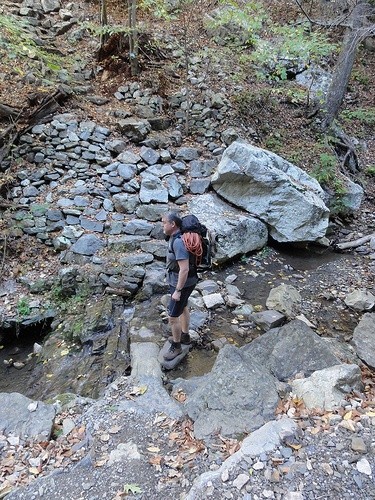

[169,214,218,273]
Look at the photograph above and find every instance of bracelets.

[175,289,181,292]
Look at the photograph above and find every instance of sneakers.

[162,346,184,361]
[167,332,192,345]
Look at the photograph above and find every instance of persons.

[162,213,199,361]
